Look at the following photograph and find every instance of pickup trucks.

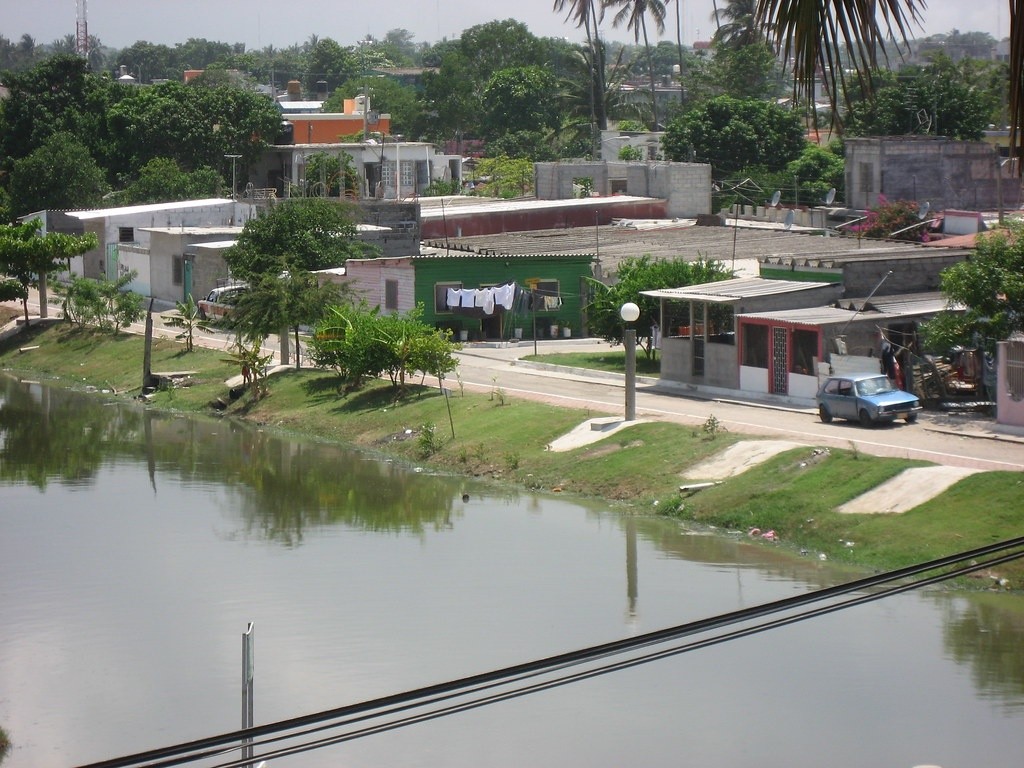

[198,286,256,329]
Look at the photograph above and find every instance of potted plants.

[562,320,572,338]
[550,319,559,338]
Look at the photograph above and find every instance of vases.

[514,328,522,339]
[459,330,468,342]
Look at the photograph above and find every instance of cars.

[816,372,923,429]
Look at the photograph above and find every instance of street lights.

[224,154,243,200]
